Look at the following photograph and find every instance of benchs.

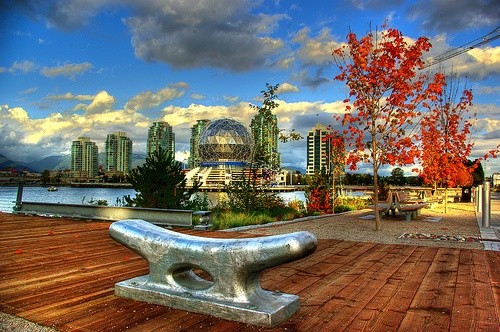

[365,192,394,218]
[392,192,428,222]
